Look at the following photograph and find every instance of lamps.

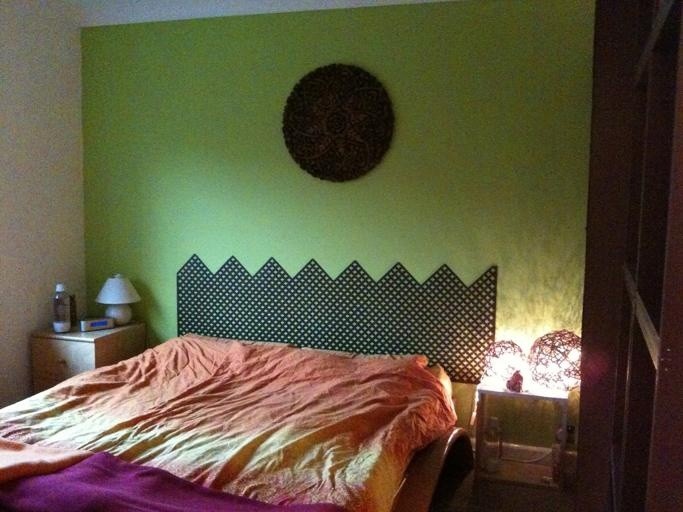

[527,327,581,391]
[483,340,526,379]
[94,274,141,326]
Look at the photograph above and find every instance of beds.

[0,255,498,512]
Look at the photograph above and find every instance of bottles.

[52,283,70,333]
[559,425,577,495]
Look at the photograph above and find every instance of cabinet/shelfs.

[576,0,683,512]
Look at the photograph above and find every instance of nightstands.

[30,323,146,395]
[474,375,571,492]
